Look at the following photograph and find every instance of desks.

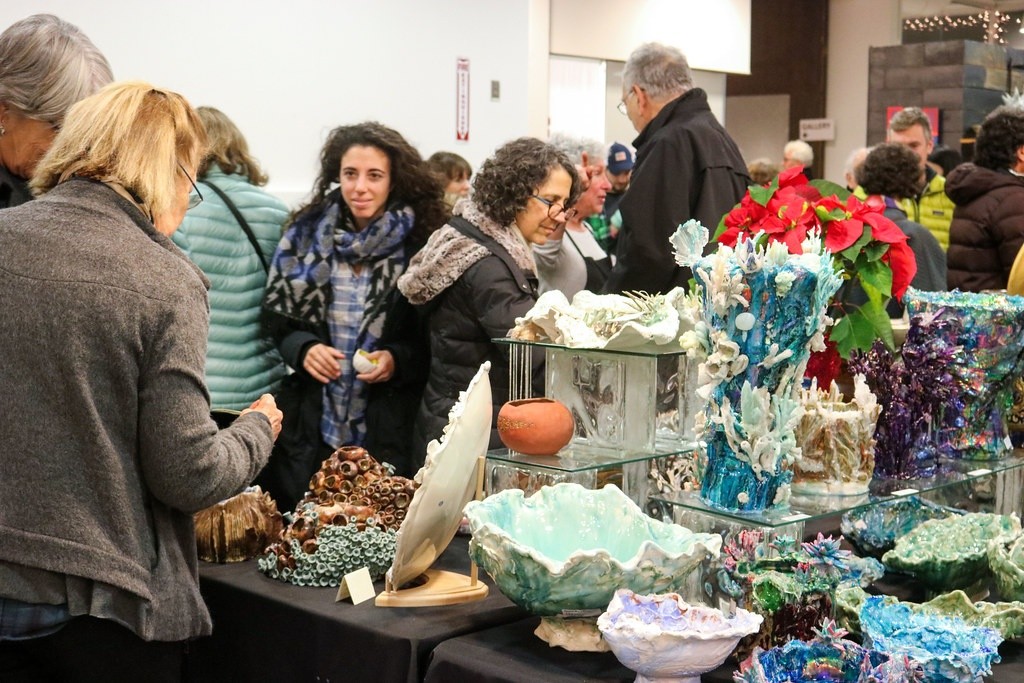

[181,513,1024,683]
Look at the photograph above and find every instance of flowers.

[722,527,924,683]
[572,219,844,511]
[688,159,917,360]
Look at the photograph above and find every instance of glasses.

[531,194,578,221]
[176,161,203,210]
[617,88,645,115]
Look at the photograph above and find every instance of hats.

[607,142,634,172]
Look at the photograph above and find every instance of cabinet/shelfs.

[486,338,695,512]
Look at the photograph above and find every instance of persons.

[397,139,581,499]
[529,134,612,302]
[167,104,293,513]
[604,143,632,218]
[0,80,284,683]
[262,122,452,514]
[0,12,113,207]
[846,88,1024,318]
[783,139,817,180]
[749,159,778,186]
[601,41,751,412]
[429,150,473,218]
